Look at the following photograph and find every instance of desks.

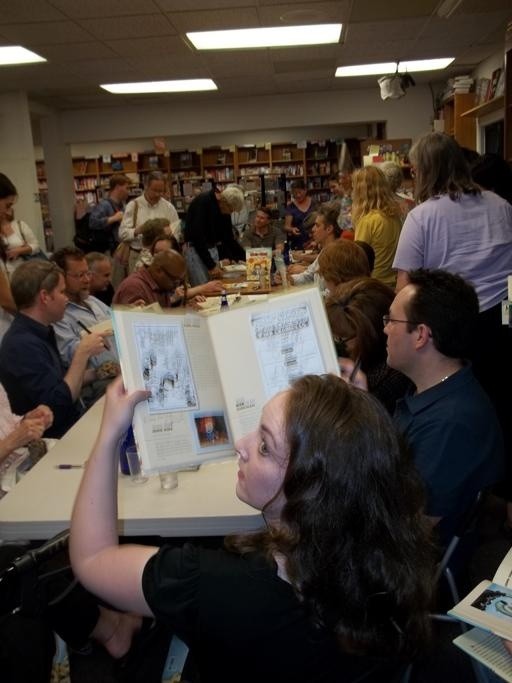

[199,274,286,293]
[1,389,266,678]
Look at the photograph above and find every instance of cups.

[126,445,149,484]
[160,474,179,491]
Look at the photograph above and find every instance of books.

[238,162,303,189]
[109,288,341,479]
[447,548,512,683]
[86,192,96,202]
[363,142,406,169]
[216,150,226,164]
[125,172,140,184]
[73,175,99,189]
[147,155,158,167]
[203,166,235,191]
[38,177,56,252]
[305,176,328,189]
[315,145,328,159]
[163,171,205,213]
[243,187,287,210]
[438,67,507,109]
[313,193,331,204]
[101,176,111,186]
[282,147,292,160]
[308,161,335,174]
[78,159,87,174]
[129,188,141,197]
[97,187,110,201]
[247,148,258,162]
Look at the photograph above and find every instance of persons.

[150,234,222,303]
[86,252,112,294]
[275,207,343,287]
[182,188,243,289]
[352,166,401,288]
[73,175,131,251]
[391,133,511,403]
[302,213,317,240]
[69,375,428,683]
[111,249,203,311]
[228,184,248,241]
[118,171,180,276]
[1,263,115,438]
[509,270,512,271]
[0,384,53,498]
[383,269,511,536]
[135,219,173,272]
[0,174,19,322]
[318,239,370,291]
[243,208,284,255]
[324,277,397,416]
[49,248,145,373]
[322,175,340,212]
[281,181,318,250]
[3,210,38,274]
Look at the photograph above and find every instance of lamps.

[380,58,415,101]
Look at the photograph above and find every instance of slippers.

[109,615,160,664]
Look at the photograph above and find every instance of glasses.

[382,313,434,338]
[334,335,356,348]
[160,267,184,285]
[66,271,91,278]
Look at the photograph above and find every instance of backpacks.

[74,212,116,251]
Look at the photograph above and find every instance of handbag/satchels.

[110,199,139,288]
[73,198,117,254]
[17,221,48,263]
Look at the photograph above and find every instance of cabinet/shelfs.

[37,138,366,249]
[457,47,511,177]
[440,93,477,151]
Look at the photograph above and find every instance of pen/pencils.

[303,248,305,253]
[349,345,370,383]
[76,319,111,352]
[220,260,223,271]
[53,464,86,470]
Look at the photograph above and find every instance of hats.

[222,188,243,213]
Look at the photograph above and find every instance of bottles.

[219,289,230,312]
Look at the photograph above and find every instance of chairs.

[430,470,499,632]
